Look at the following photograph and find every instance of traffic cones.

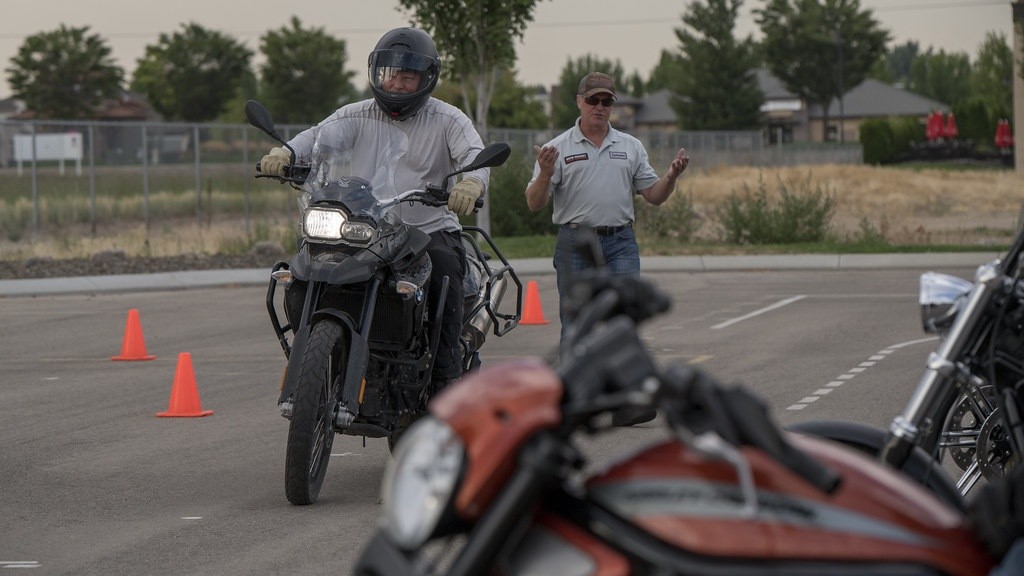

[518,280,549,325]
[155,352,216,418]
[112,309,157,361]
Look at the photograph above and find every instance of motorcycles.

[243,97,524,507]
[882,224,1023,501]
[346,208,1022,576]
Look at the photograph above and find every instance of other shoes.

[611,404,657,426]
[432,364,461,394]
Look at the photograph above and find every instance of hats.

[578,71,619,100]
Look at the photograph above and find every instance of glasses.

[584,96,615,106]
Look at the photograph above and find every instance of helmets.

[368,26,442,122]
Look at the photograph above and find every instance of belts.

[560,222,632,236]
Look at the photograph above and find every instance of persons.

[526,70,689,364]
[263,25,490,401]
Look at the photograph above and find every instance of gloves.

[262,147,292,180]
[447,176,483,217]
